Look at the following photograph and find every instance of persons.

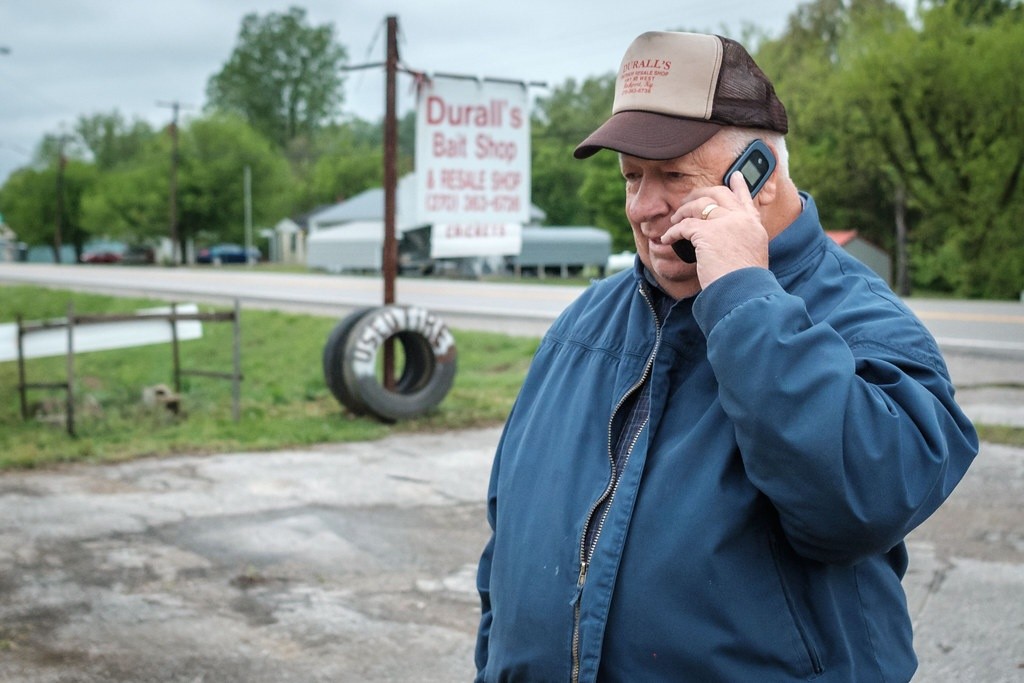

[474,30,980,683]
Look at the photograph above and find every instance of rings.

[701,204,720,221]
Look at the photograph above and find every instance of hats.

[573,30,790,161]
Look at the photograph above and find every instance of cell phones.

[674,140,777,263]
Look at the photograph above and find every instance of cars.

[196,242,263,267]
[79,239,155,265]
